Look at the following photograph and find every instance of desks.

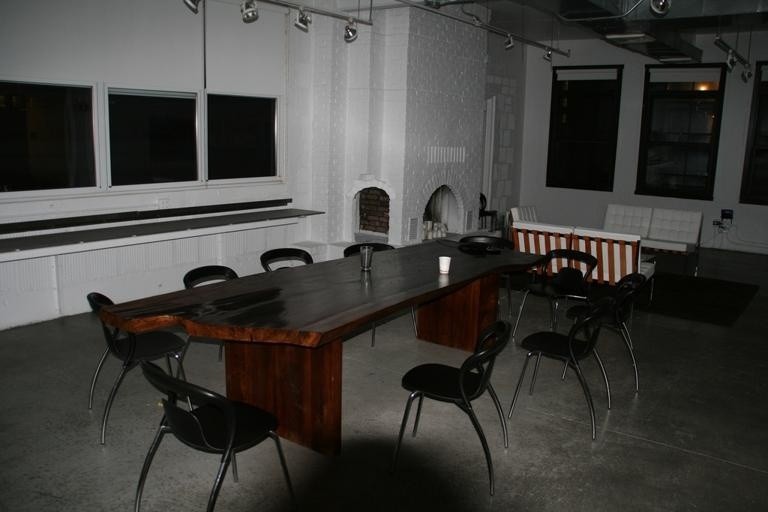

[101,241,544,463]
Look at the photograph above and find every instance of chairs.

[566,273,647,391]
[480,193,498,228]
[571,227,657,336]
[344,243,418,346]
[86,292,185,445]
[260,248,313,271]
[180,266,239,360]
[512,250,597,335]
[135,360,293,512]
[510,206,539,222]
[509,307,612,440]
[512,221,576,319]
[603,203,704,276]
[460,236,515,249]
[396,320,509,496]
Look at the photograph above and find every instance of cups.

[439,275,450,286]
[361,270,372,289]
[439,256,451,273]
[360,246,373,268]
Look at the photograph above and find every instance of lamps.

[499,31,570,63]
[182,0,372,43]
[649,0,671,18]
[712,34,752,83]
